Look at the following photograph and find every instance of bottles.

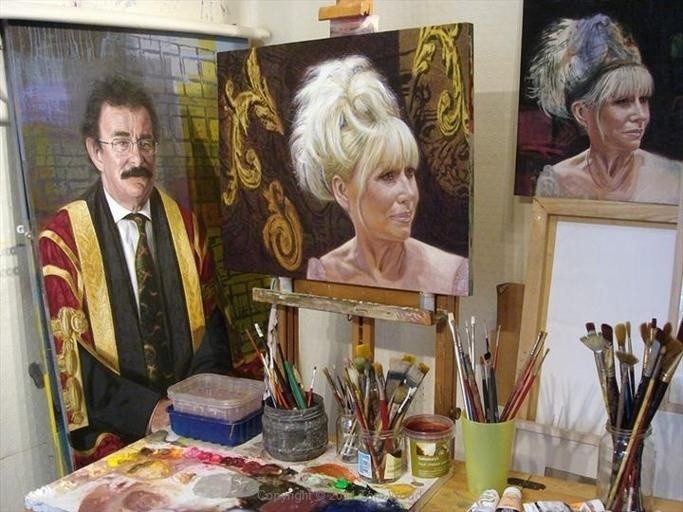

[260,390,328,461]
[595,421,655,511]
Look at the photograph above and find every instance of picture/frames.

[515,196,683,423]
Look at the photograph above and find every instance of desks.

[26,426,683,512]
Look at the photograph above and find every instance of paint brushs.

[246,322,320,411]
[580,319,683,511]
[446,310,551,424]
[323,345,429,484]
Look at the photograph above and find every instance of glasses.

[96,134,160,157]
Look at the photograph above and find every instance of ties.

[121,214,173,389]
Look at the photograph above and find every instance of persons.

[525,16,682,206]
[39,73,231,467]
[288,55,468,296]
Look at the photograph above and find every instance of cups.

[337,411,454,483]
[460,409,516,494]
